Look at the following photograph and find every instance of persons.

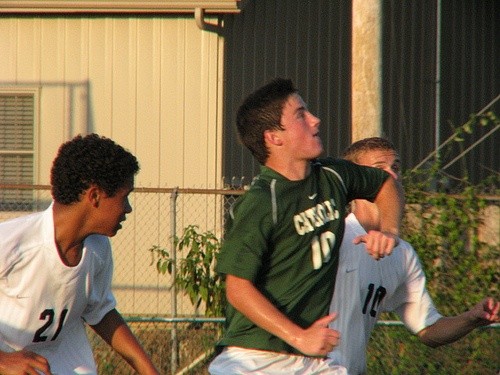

[0,133,160,375]
[206,77,406,375]
[325,137,500,375]
